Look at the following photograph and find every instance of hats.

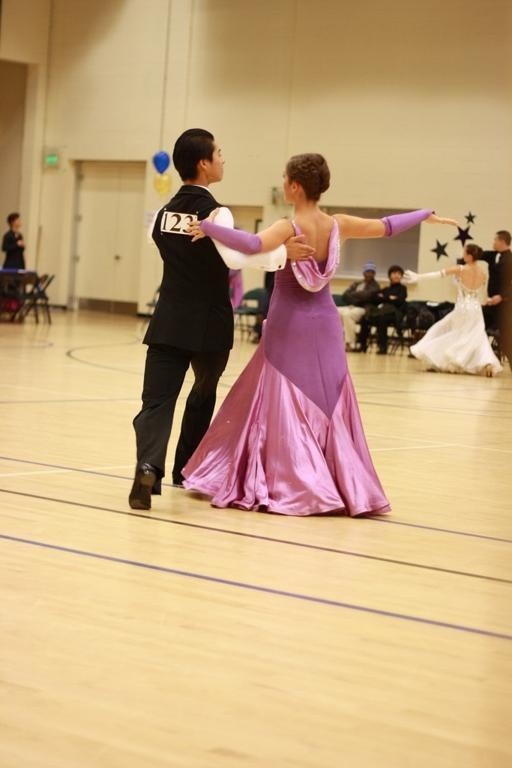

[362,261,375,273]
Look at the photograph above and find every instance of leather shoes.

[377,350,387,354]
[357,344,368,352]
[130,464,155,510]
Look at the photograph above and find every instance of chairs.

[0,269,55,324]
[143,287,506,361]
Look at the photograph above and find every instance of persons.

[355,265,408,358]
[180,149,460,520]
[479,231,511,371]
[0,211,27,294]
[398,242,503,379]
[334,259,383,352]
[123,123,320,512]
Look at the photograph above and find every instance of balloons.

[153,172,175,196]
[151,150,172,175]
